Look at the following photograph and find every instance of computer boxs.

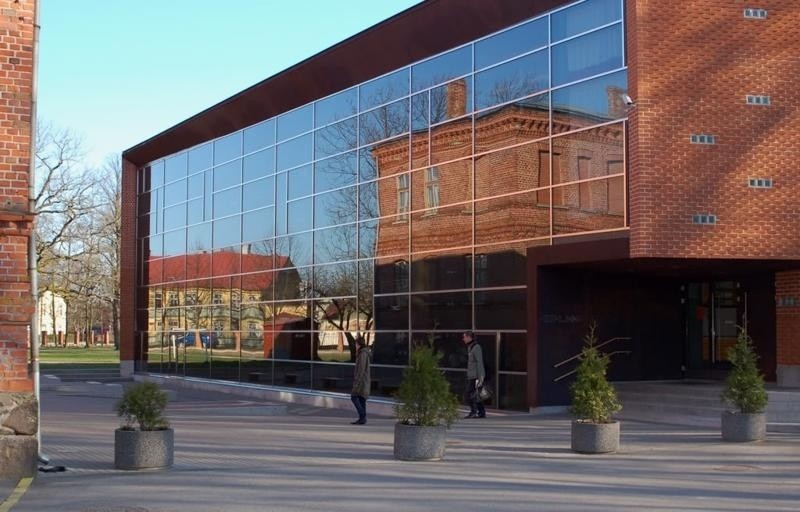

[622,94,632,106]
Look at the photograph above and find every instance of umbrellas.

[464,410,487,418]
[349,417,366,424]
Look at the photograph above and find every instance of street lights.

[474,381,496,404]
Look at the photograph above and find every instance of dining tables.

[570,320,623,455]
[720,318,770,441]
[113,383,176,469]
[394,325,461,462]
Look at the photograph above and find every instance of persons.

[463,330,487,420]
[350,336,373,424]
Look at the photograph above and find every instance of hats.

[176,332,218,348]
